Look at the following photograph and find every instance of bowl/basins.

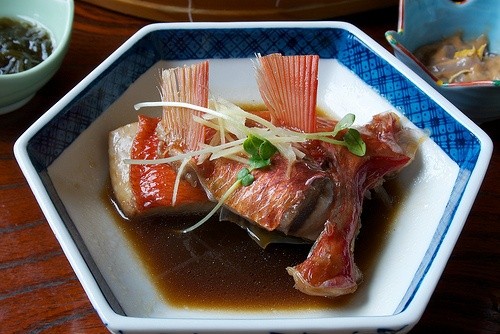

[0,0,75,114]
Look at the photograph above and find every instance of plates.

[385,0,500,124]
[13,20,496,334]
